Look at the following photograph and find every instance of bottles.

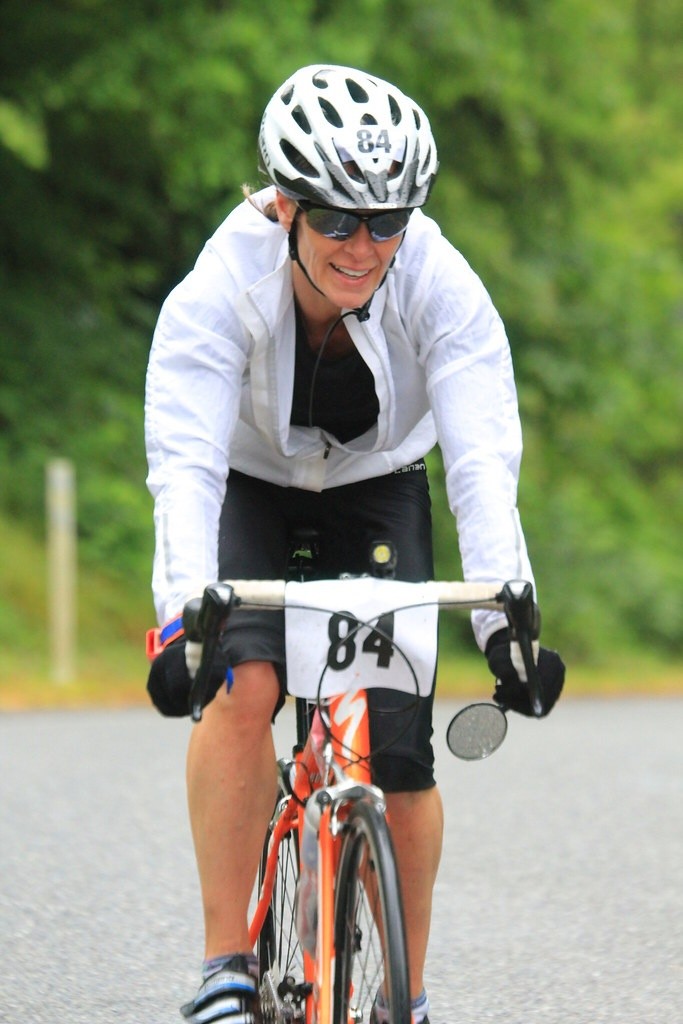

[296,788,342,951]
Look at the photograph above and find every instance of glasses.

[294,200,413,243]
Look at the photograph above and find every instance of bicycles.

[183,578,547,1024]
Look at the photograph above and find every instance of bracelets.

[146,611,210,656]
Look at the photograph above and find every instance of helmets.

[257,65,440,209]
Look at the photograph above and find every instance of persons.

[145,64,566,1024]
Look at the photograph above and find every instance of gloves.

[485,626,566,717]
[147,634,226,717]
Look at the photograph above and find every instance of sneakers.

[370,981,431,1024]
[179,952,259,1024]
